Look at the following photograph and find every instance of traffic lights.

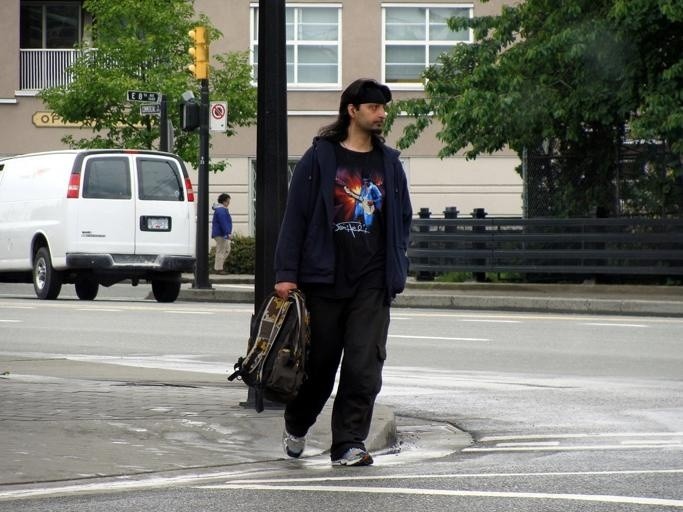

[180,27,209,131]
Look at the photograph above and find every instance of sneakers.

[282,426,306,458]
[330,447,373,467]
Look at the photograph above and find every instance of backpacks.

[227,287,312,414]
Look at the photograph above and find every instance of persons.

[211,193,233,276]
[272,77,413,466]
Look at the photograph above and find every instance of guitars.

[344,186,375,215]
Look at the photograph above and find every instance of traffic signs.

[127,91,161,115]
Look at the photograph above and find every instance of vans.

[0,149,197,302]
